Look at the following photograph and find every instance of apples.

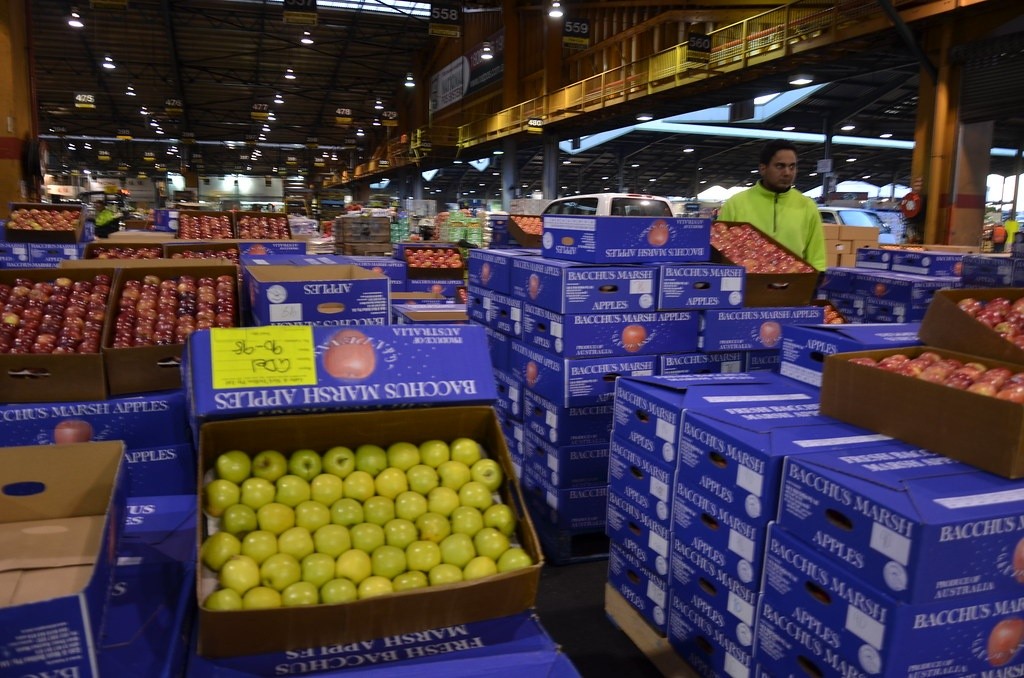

[821,304,843,324]
[405,249,467,304]
[0,208,288,353]
[411,235,420,242]
[510,215,543,235]
[848,294,1024,404]
[707,222,814,273]
[201,436,534,611]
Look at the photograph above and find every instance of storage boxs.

[0,198,1024,678]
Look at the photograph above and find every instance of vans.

[540,192,674,217]
[818,207,895,244]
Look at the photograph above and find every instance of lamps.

[356,128,365,136]
[140,106,149,114]
[481,41,493,59]
[274,91,285,104]
[126,82,137,97]
[375,99,384,109]
[268,110,277,121]
[69,121,269,172]
[547,0,565,18]
[331,153,338,160]
[68,7,85,28]
[405,73,415,87]
[301,30,314,45]
[285,68,296,80]
[373,117,381,125]
[322,151,330,157]
[102,52,116,69]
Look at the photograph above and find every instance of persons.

[989,223,1007,253]
[251,204,274,210]
[94,200,112,226]
[719,139,825,279]
